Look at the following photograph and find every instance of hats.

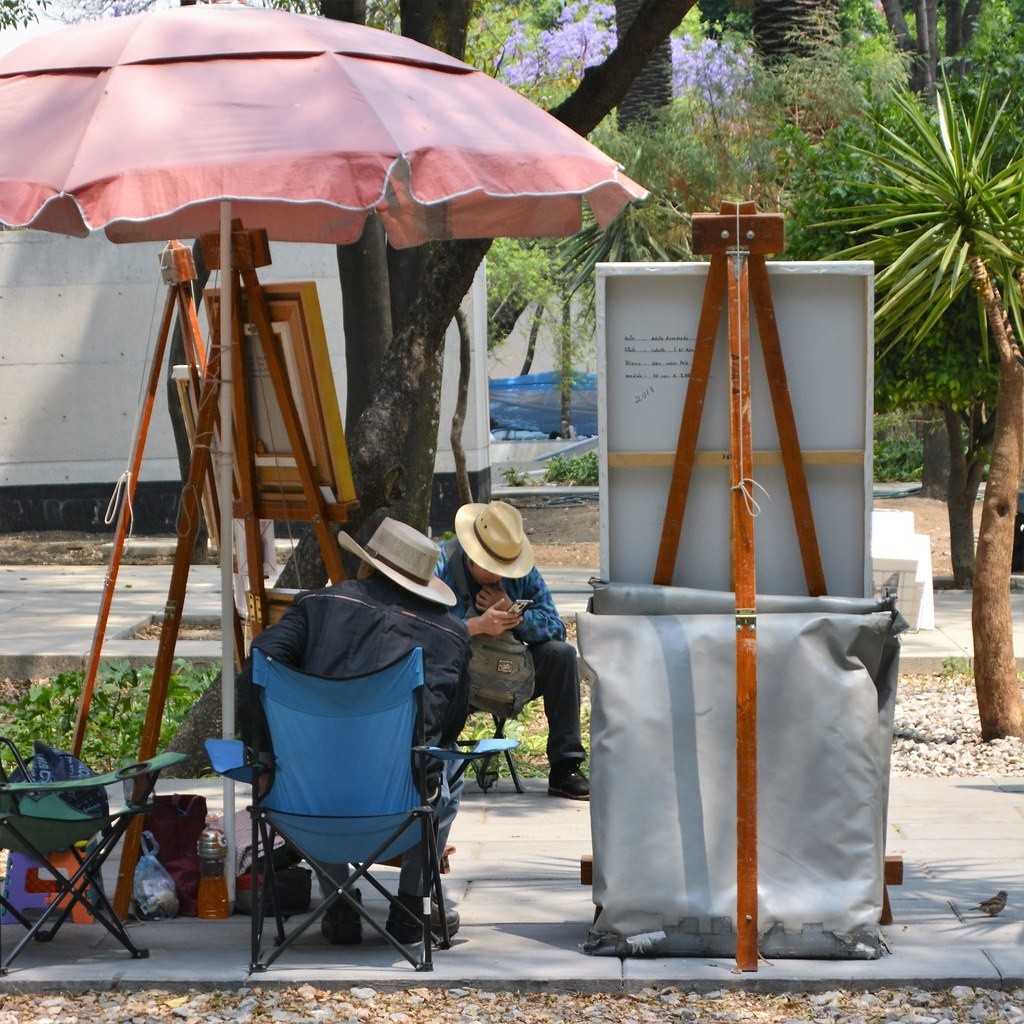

[337,517,457,607]
[455,501,535,579]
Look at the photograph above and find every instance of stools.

[0,833,107,925]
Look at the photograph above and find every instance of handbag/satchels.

[7,741,111,819]
[133,830,179,921]
[131,794,207,921]
[463,607,536,718]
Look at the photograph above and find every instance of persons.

[413,496,593,800]
[242,517,461,944]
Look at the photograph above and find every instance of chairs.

[0,734,194,976]
[204,646,525,973]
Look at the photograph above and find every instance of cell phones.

[506,599,534,617]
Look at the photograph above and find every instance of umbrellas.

[0,0,653,897]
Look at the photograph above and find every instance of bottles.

[195,813,231,920]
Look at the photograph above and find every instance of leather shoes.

[381,896,461,945]
[322,890,362,946]
[547,763,590,799]
